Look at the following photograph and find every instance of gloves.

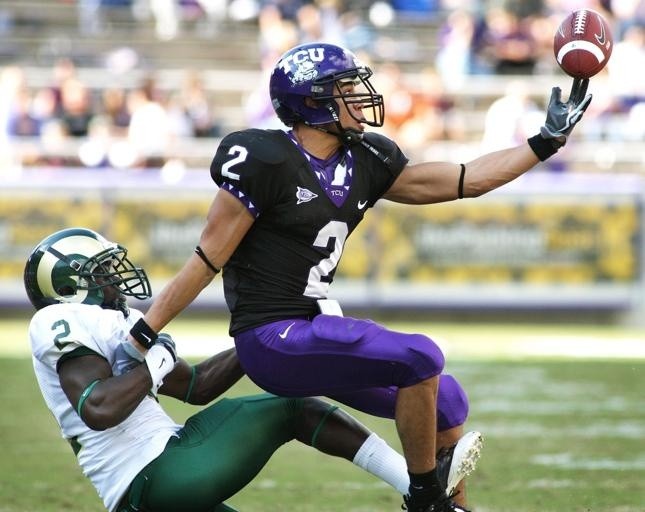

[154,333,178,369]
[111,339,145,377]
[540,76,593,145]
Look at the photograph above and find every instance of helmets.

[269,42,384,128]
[23,228,152,311]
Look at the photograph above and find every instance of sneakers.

[401,488,470,512]
[403,431,484,503]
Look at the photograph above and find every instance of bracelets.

[127,319,158,350]
[145,344,174,390]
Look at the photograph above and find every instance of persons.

[112,44,594,512]
[1,0,644,181]
[23,227,483,512]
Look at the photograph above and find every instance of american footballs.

[554,9,613,79]
